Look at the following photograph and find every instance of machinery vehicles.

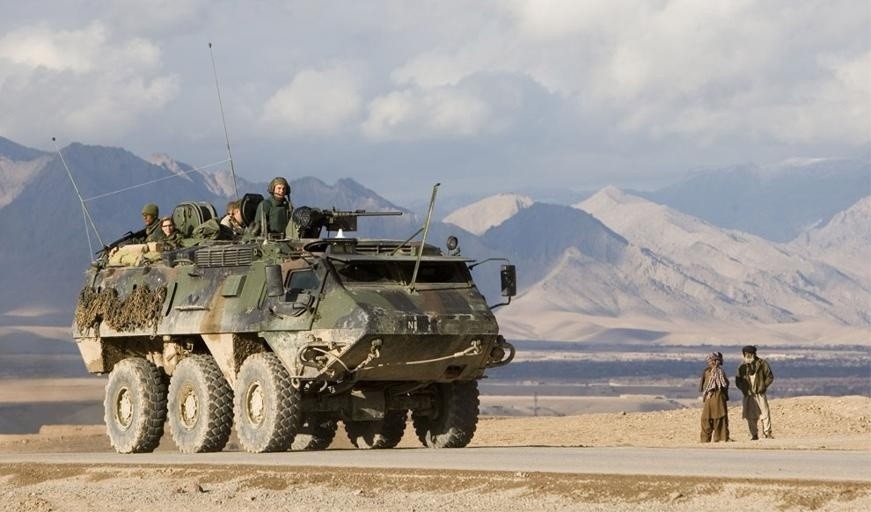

[52,44,516,453]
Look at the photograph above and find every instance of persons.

[734,345,773,439]
[699,351,734,444]
[126,203,163,242]
[158,217,184,252]
[220,176,294,242]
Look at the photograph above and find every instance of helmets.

[141,203,159,216]
